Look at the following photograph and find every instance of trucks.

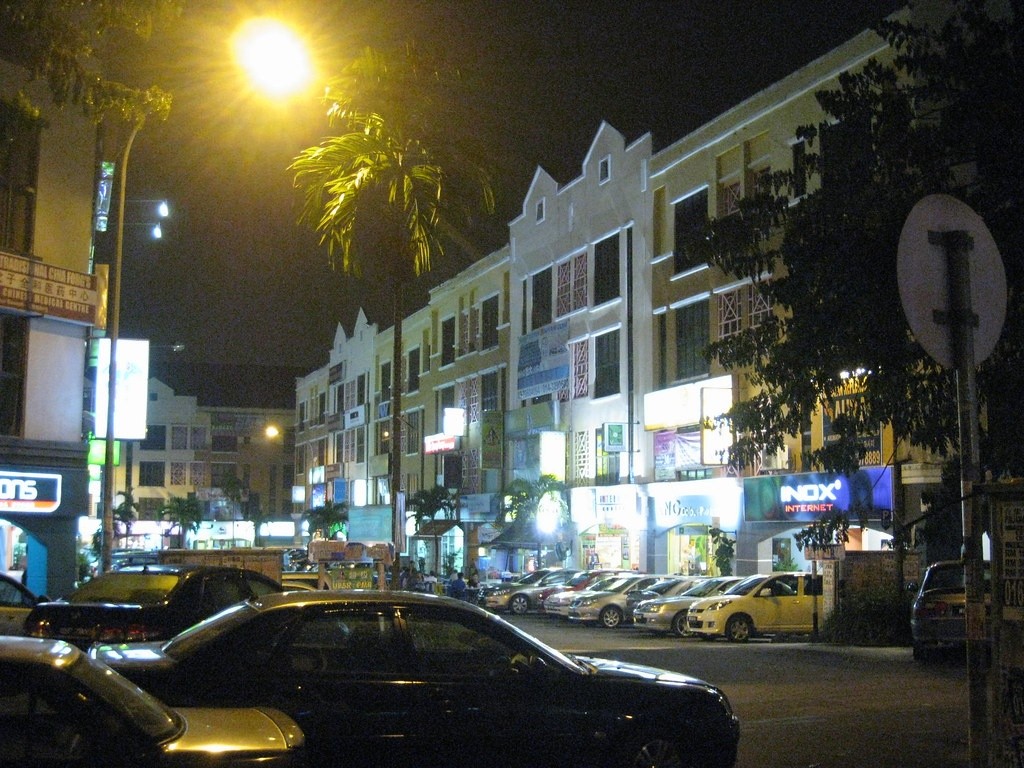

[114,546,284,589]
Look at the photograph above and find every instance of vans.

[282,574,333,592]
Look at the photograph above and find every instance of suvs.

[537,569,636,614]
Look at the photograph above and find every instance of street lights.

[265,426,314,536]
[100,12,327,569]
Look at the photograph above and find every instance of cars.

[1,633,307,768]
[82,587,741,768]
[23,563,285,649]
[568,575,679,629]
[625,577,712,618]
[684,571,823,642]
[484,567,583,615]
[284,545,317,571]
[544,576,638,616]
[908,558,996,662]
[632,575,744,639]
[0,571,51,633]
[282,582,316,592]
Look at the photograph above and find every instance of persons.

[450,570,458,581]
[20,555,28,584]
[467,574,480,588]
[399,560,438,589]
[453,573,467,597]
